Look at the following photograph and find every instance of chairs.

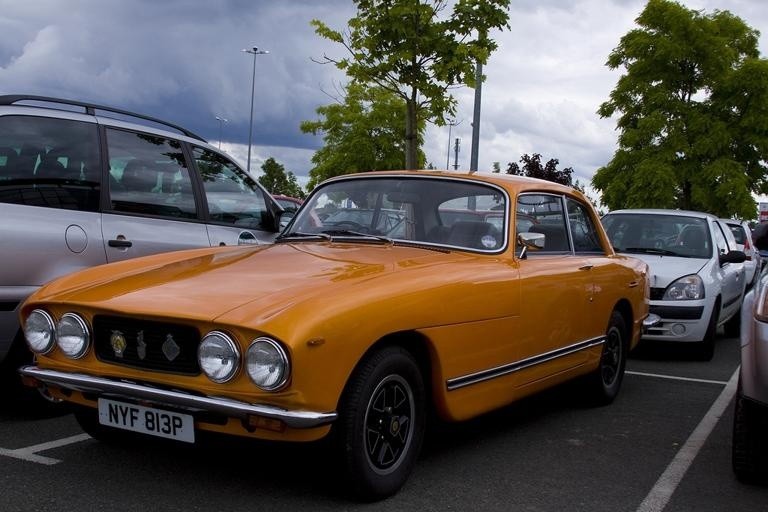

[526,223,575,251]
[442,220,497,251]
[121,157,159,193]
[678,225,712,256]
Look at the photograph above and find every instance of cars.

[730,223,768,490]
[13,170,654,497]
[571,210,748,362]
[675,218,761,288]
[189,192,542,242]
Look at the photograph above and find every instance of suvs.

[0,96,306,365]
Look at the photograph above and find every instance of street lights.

[239,45,270,172]
[215,111,228,150]
[453,137,462,171]
